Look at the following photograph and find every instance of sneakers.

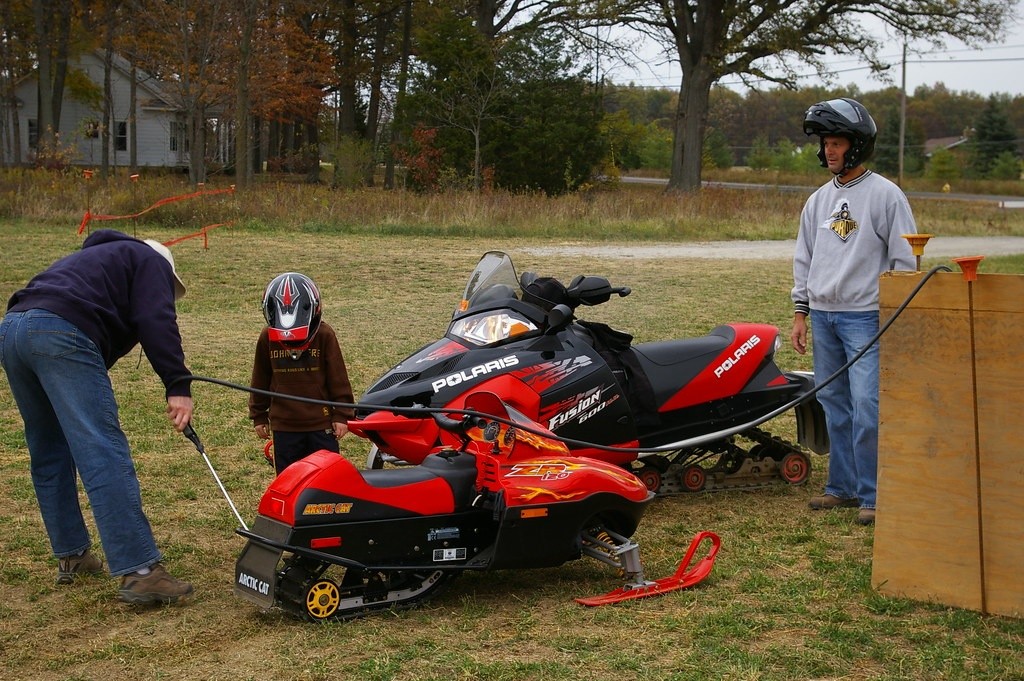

[809,494,859,509]
[57,549,103,584]
[118,566,193,602]
[858,508,875,521]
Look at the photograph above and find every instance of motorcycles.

[355,250,827,488]
[229,385,657,622]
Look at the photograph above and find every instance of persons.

[791,98,917,523]
[0,230,193,605]
[249,272,355,477]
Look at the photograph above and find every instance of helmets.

[803,97,877,169]
[262,272,322,360]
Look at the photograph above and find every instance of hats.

[143,240,187,300]
